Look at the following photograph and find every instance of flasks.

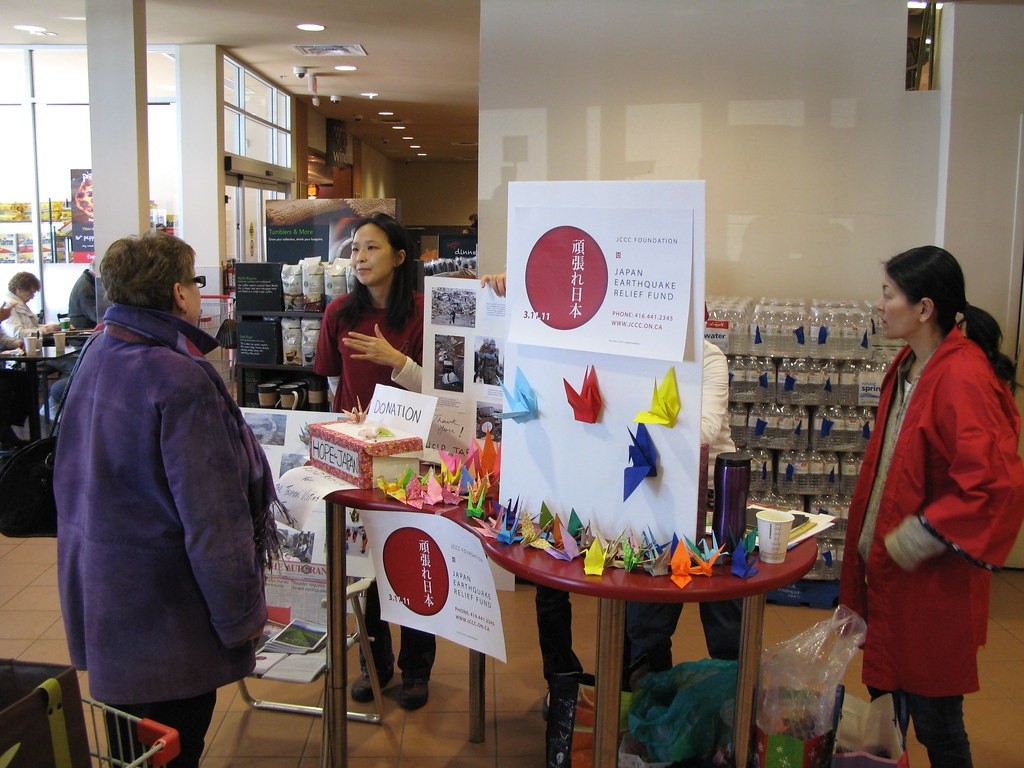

[712,452,753,555]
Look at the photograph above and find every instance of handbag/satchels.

[546,670,908,768]
[0,435,57,538]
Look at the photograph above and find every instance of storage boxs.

[308,419,424,490]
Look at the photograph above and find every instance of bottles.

[307,371,324,412]
[704,294,911,580]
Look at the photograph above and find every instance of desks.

[0,346,80,439]
[323,487,818,768]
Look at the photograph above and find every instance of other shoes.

[39,403,62,421]
[351,671,392,703]
[542,692,550,721]
[399,679,429,711]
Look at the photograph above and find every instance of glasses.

[184,275,206,288]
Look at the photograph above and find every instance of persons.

[449,309,456,324]
[69,258,96,331]
[1,272,61,340]
[625,300,742,695]
[53,228,299,768]
[313,210,437,709]
[834,243,1024,768]
[474,338,503,383]
[0,301,30,450]
[483,272,628,712]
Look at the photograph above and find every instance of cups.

[756,510,795,564]
[60,318,71,332]
[24,337,36,356]
[36,337,43,349]
[257,374,307,411]
[53,333,66,349]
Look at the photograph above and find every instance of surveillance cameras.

[354,115,363,121]
[382,138,389,144]
[293,67,307,78]
[330,96,342,104]
